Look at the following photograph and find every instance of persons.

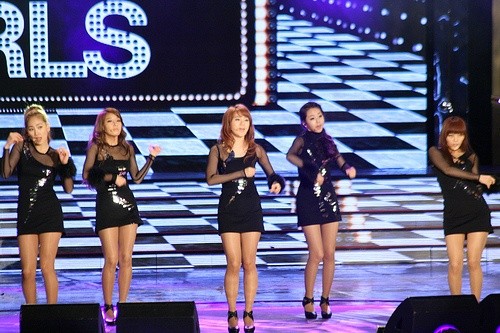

[83,109,159,325]
[206,104,285,333]
[286,102,357,319]
[430,117,495,300]
[0,104,76,305]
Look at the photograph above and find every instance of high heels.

[302,296,317,319]
[320,296,332,318]
[242,310,255,333]
[227,310,240,333]
[103,303,115,325]
